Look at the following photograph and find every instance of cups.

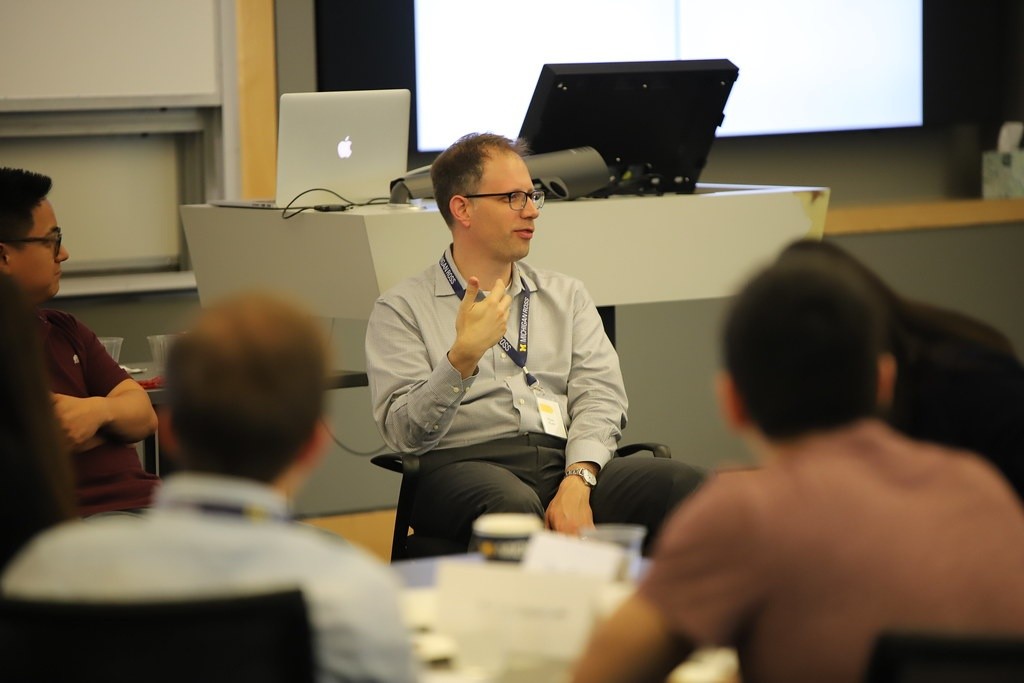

[146,334,180,376]
[472,511,544,563]
[97,336,124,364]
[580,523,647,586]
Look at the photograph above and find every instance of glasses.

[463,189,546,211]
[2,226,62,257]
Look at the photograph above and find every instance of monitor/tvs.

[515,58,739,196]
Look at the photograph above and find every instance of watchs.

[564,467,597,489]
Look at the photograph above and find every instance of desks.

[118,358,369,480]
[178,183,831,527]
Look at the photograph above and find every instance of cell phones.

[313,205,346,212]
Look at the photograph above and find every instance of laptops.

[207,89,411,210]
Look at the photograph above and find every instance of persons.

[365,133,708,559]
[571,262,1024,683]
[1,295,423,682]
[0,166,163,514]
[784,237,1024,504]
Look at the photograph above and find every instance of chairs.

[371,443,673,564]
[1,584,314,683]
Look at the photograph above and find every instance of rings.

[580,536,587,540]
